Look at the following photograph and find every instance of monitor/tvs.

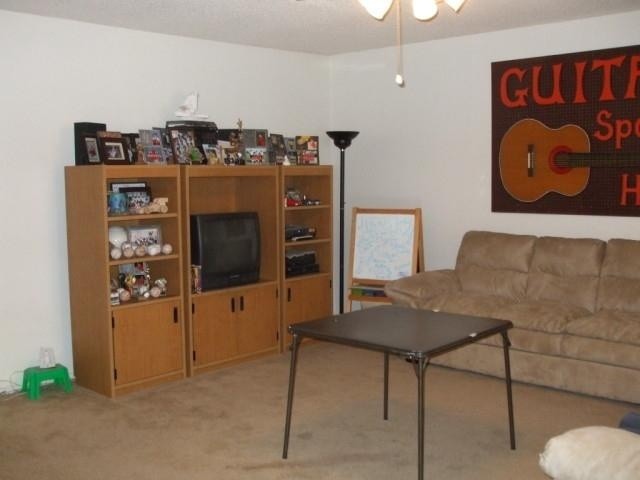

[191,212,261,291]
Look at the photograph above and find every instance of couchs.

[384,230,640,405]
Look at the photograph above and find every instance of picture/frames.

[100,137,129,165]
[81,134,102,164]
[244,147,269,165]
[255,129,268,147]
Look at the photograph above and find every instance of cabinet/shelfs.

[280,165,334,354]
[63,163,186,400]
[182,163,280,377]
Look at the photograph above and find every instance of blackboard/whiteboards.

[348,205,425,285]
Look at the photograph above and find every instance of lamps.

[326,131,360,316]
[358,0,466,86]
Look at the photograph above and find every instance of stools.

[22,364,73,400]
